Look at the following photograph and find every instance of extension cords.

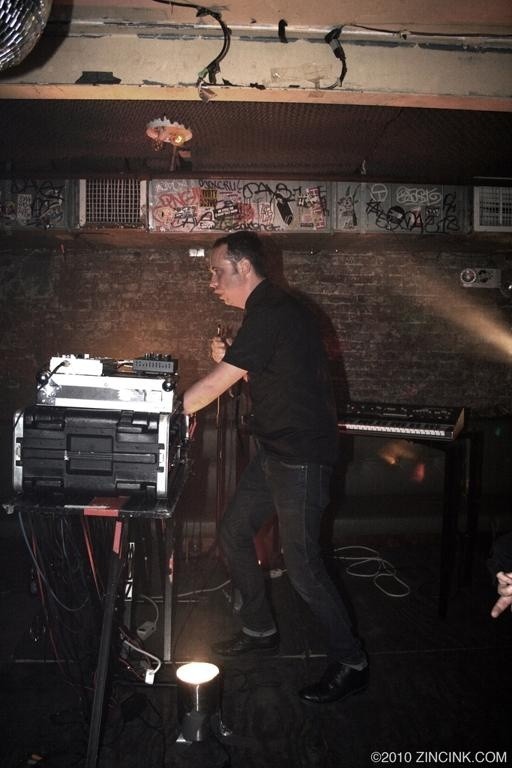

[269,568,282,578]
[137,621,157,640]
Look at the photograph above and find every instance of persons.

[492,570,512,620]
[184,231,377,702]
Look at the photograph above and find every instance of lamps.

[174,660,229,741]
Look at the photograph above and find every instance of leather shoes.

[209,629,282,658]
[295,660,374,705]
